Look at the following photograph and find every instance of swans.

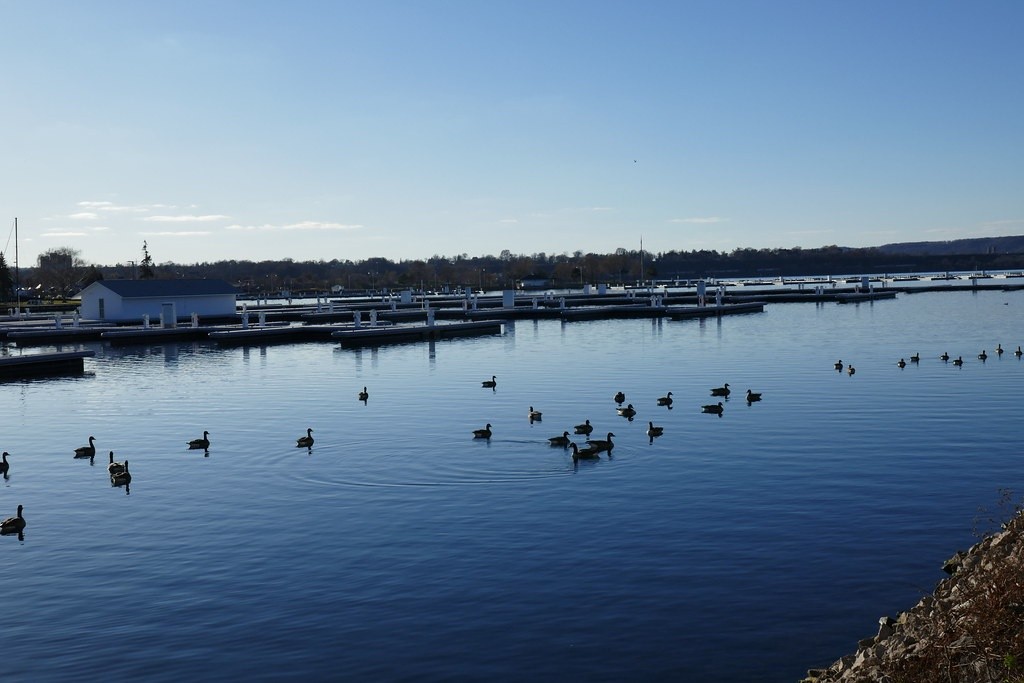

[1,344,1024,535]
